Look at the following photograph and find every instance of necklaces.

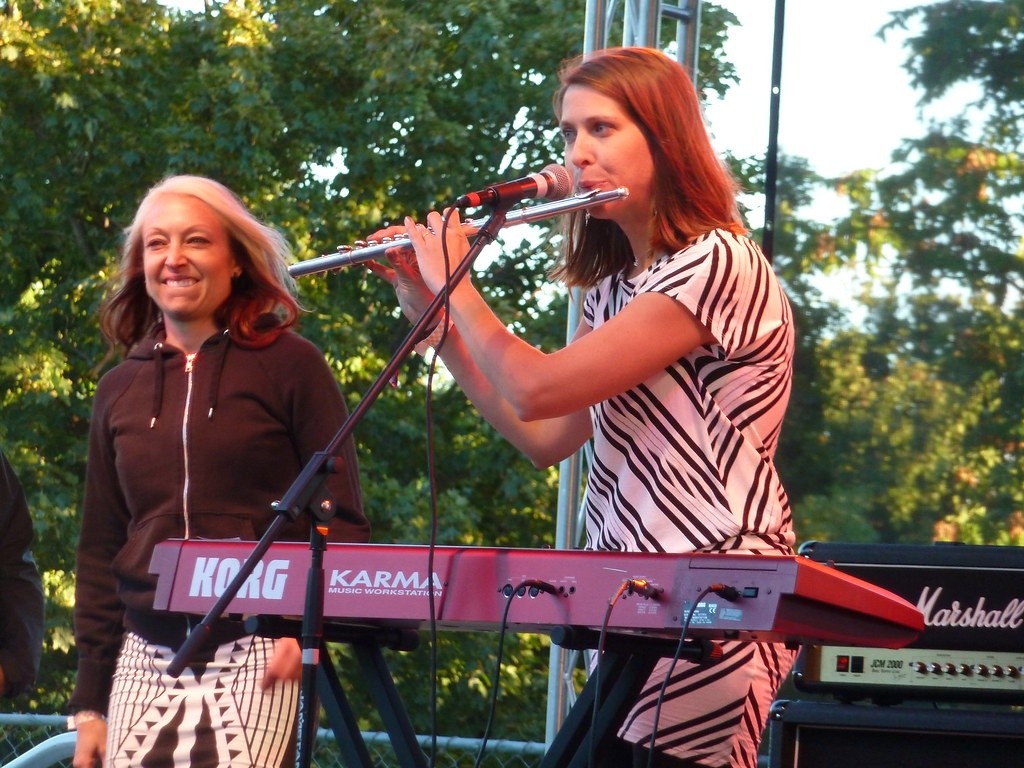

[633,247,654,267]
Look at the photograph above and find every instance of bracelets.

[414,312,454,358]
[67,710,103,731]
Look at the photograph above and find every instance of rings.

[421,231,432,238]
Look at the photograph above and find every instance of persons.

[54,175,369,768]
[366,48,802,768]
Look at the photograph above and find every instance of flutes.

[286,187,630,278]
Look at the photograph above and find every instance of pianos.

[147,533,925,767]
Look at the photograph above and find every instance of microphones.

[457,163,572,208]
